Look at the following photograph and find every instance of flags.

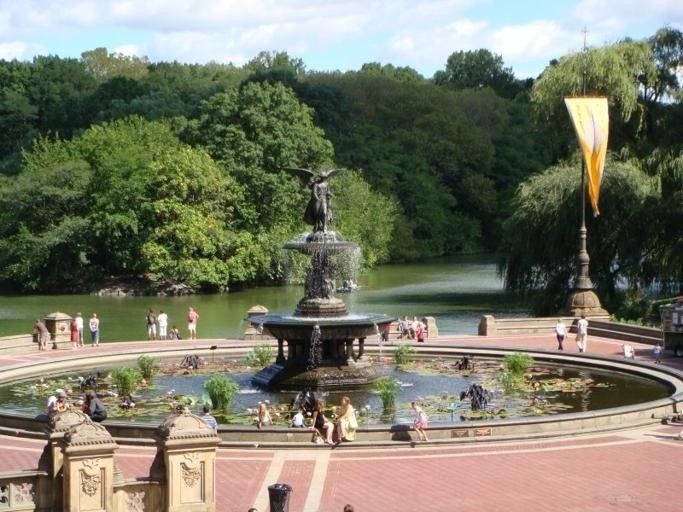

[561,97,609,218]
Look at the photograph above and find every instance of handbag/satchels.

[313,431,324,443]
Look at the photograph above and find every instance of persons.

[311,172,334,234]
[201,405,218,437]
[335,396,358,443]
[344,504,354,512]
[555,318,568,350]
[70,311,100,348]
[575,314,589,352]
[346,277,353,292]
[308,398,335,445]
[248,508,258,512]
[381,313,425,343]
[653,341,663,364]
[258,403,274,425]
[411,401,428,441]
[291,411,305,428]
[33,318,49,351]
[46,388,107,422]
[145,306,199,339]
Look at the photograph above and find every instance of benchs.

[384,322,428,341]
